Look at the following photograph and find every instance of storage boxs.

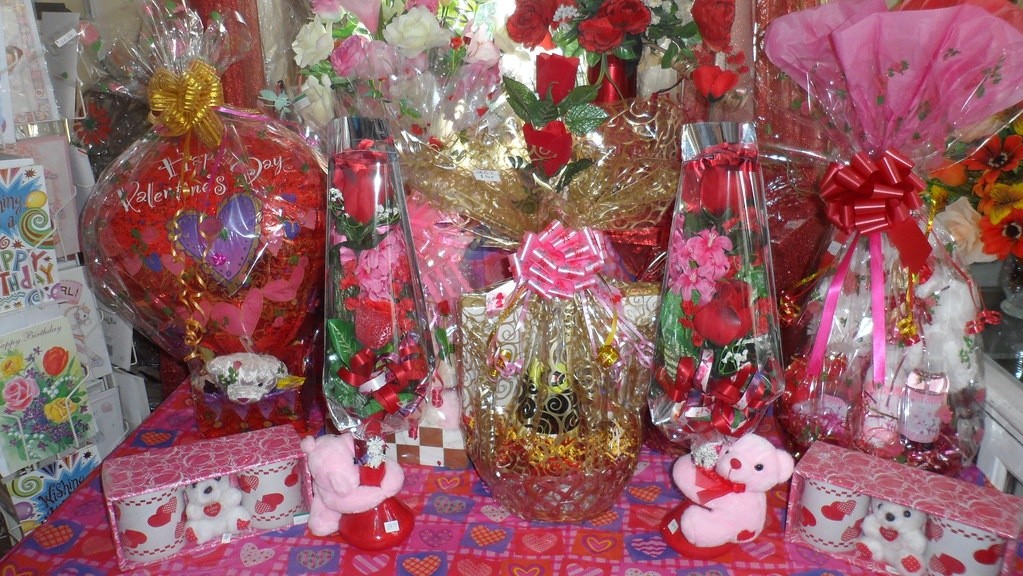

[784,440,1023,575]
[101,422,309,572]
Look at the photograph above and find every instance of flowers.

[74,0,1023,469]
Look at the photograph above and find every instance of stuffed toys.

[854,233,989,459]
[299,432,405,538]
[661,434,795,547]
[180,474,251,544]
[205,351,289,406]
[855,496,931,575]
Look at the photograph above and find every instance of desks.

[1,369,1002,575]
